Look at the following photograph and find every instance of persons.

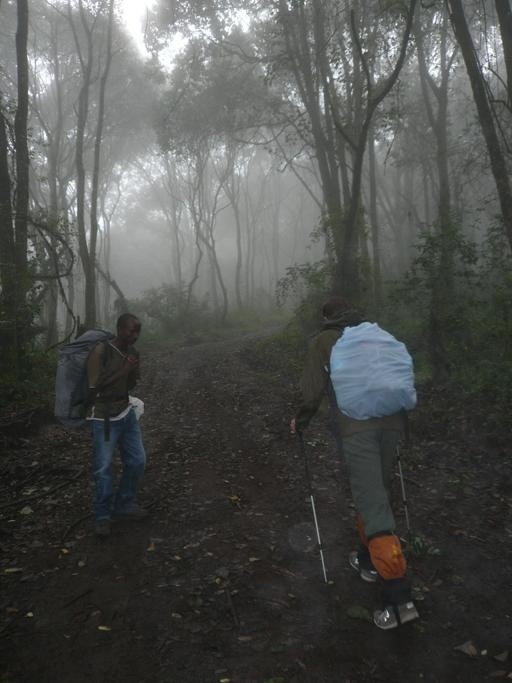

[82,312,155,537]
[288,297,419,628]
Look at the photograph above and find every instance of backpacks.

[316,323,418,420]
[54,329,116,430]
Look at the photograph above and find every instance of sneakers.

[372,601,418,631]
[95,518,111,535]
[349,551,378,582]
